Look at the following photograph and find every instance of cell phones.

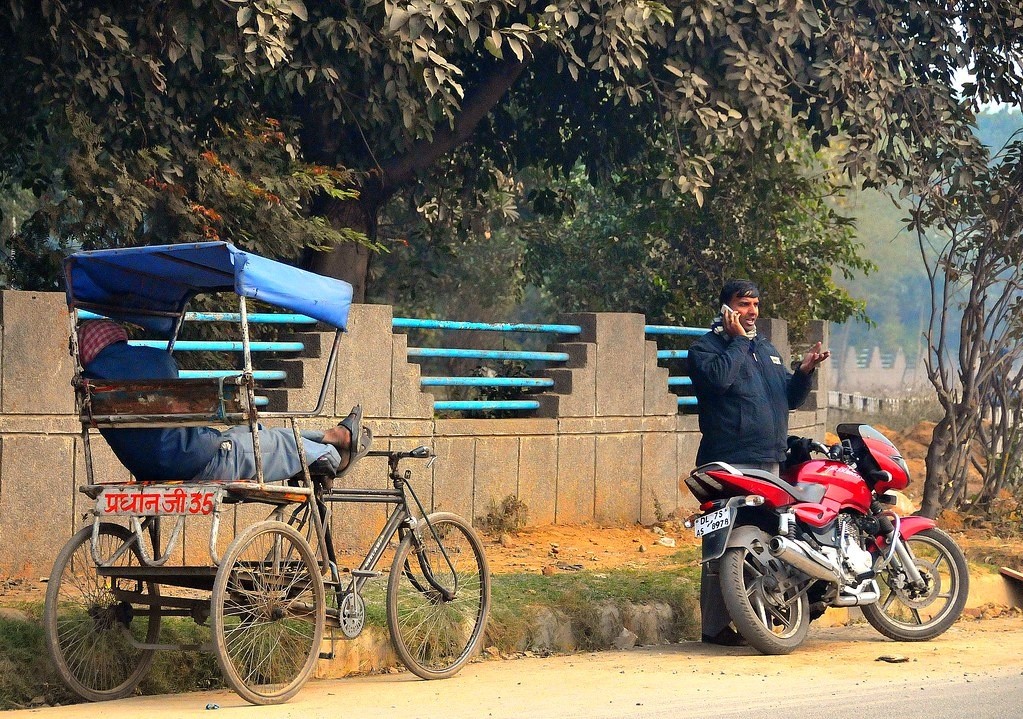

[721,303,733,316]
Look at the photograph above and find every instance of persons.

[686,281,830,649]
[67,318,372,483]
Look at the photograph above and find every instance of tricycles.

[44,243,491,706]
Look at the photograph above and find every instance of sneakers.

[701,626,748,647]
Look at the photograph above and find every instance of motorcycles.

[682,423,970,656]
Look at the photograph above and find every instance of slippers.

[329,428,374,478]
[338,402,365,453]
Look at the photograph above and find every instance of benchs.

[67,374,330,574]
